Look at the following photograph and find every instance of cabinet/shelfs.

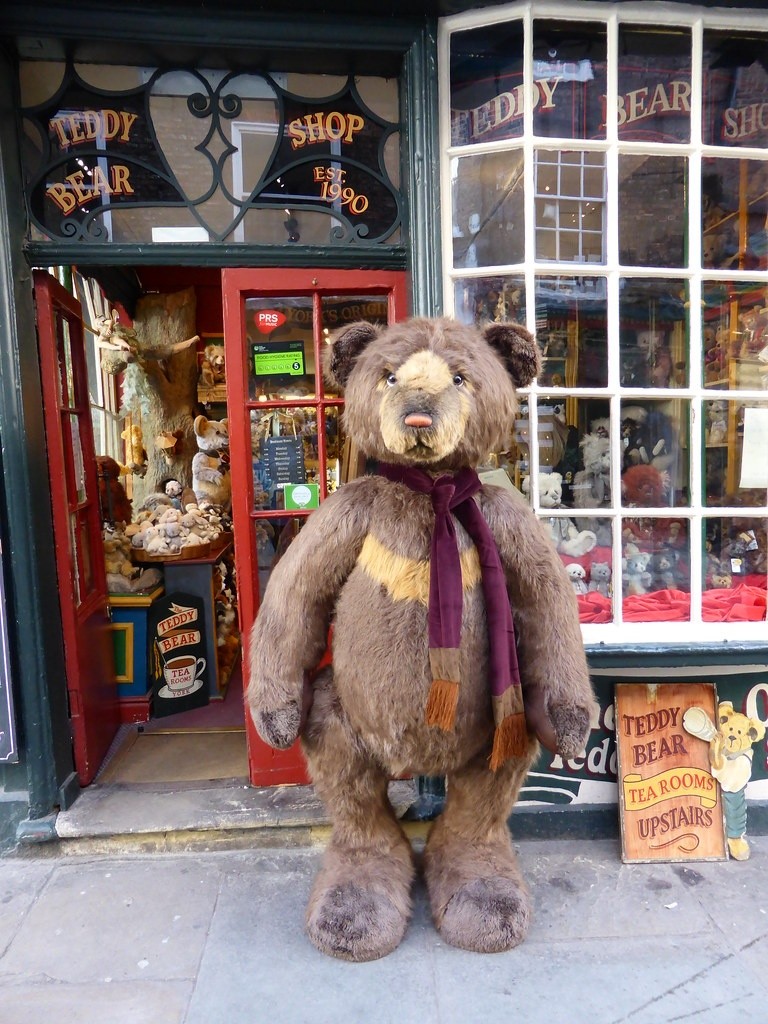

[680,296,767,500]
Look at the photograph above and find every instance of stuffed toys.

[95,456,133,526]
[248,385,341,566]
[701,233,731,270]
[199,343,226,386]
[590,561,610,597]
[705,541,719,573]
[672,315,730,388]
[192,414,232,514]
[618,466,685,548]
[723,537,751,576]
[636,330,672,387]
[703,400,729,444]
[721,488,768,574]
[650,551,689,593]
[213,555,240,685]
[589,405,677,469]
[522,472,596,557]
[618,344,647,388]
[102,492,233,555]
[710,568,732,589]
[731,306,768,364]
[575,432,624,546]
[101,520,136,577]
[705,447,727,496]
[536,327,566,388]
[565,563,589,595]
[116,425,144,477]
[248,318,597,958]
[624,552,651,595]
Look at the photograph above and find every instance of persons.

[515,409,583,506]
